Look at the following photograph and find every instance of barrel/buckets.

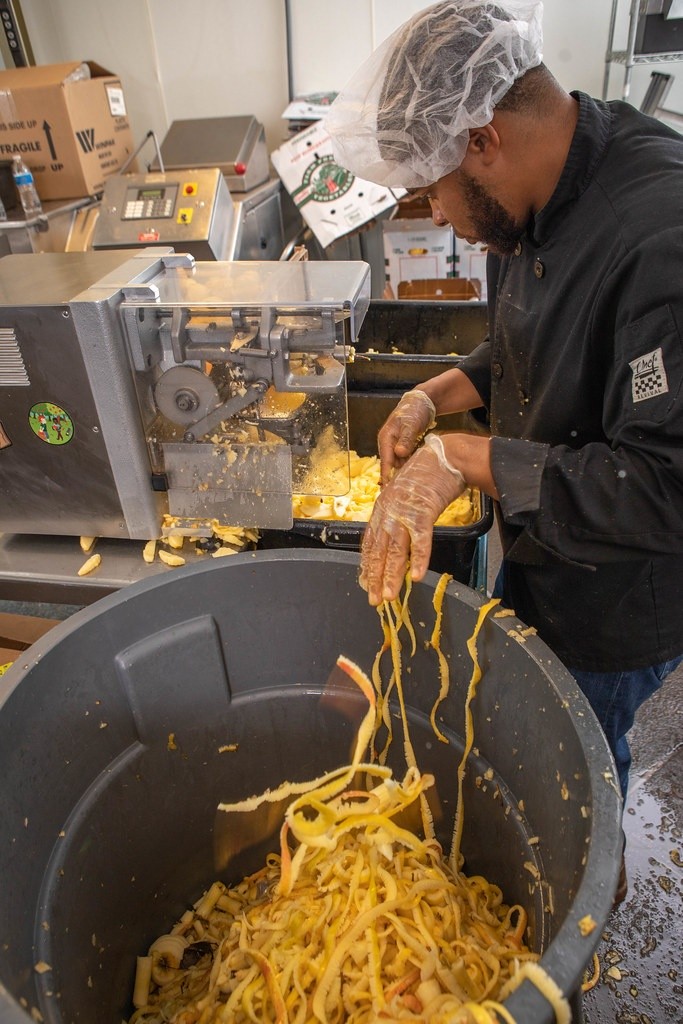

[0,548,624,1023]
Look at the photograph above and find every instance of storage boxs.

[0,58,141,205]
[261,375,496,599]
[275,81,496,304]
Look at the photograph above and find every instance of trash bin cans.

[0,548,623,1024]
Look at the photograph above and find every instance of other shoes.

[612,854,629,904]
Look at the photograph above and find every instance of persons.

[324,0,683,901]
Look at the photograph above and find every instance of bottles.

[12,153,43,213]
